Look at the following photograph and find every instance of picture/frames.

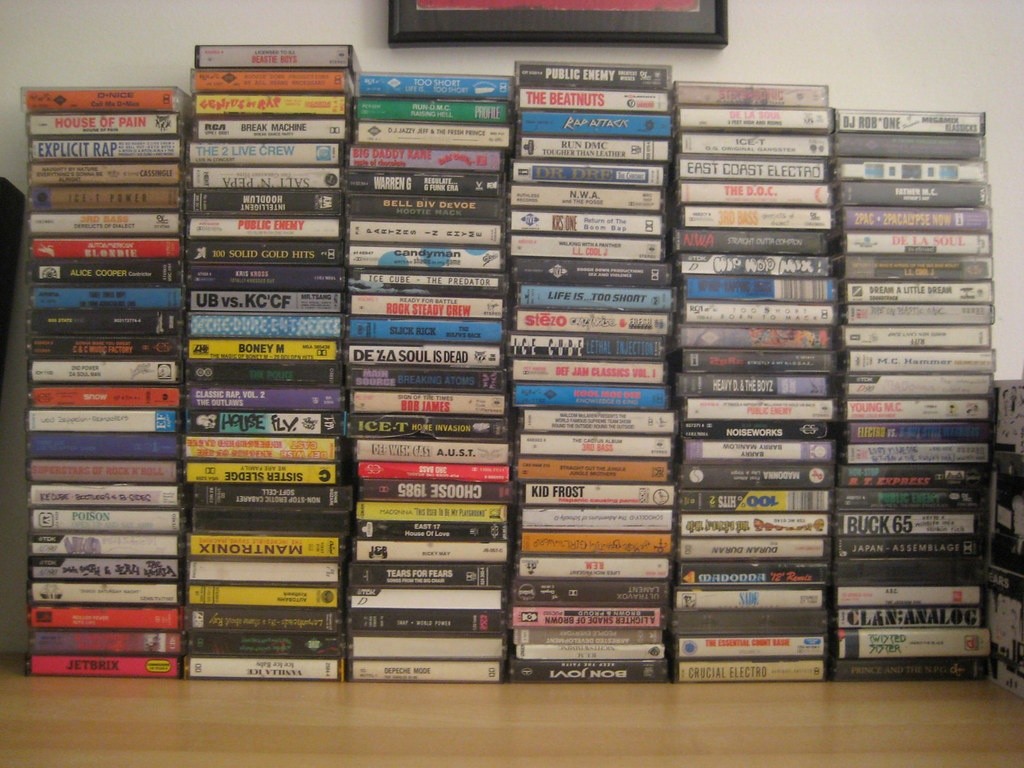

[387,0,729,50]
